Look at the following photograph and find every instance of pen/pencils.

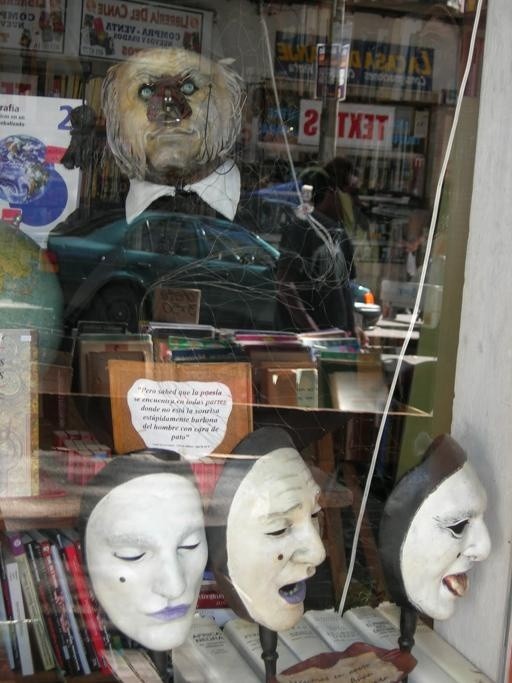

[235,336,298,341]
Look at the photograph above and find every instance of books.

[151,283,202,325]
[1,315,396,499]
[1,70,127,250]
[101,600,495,683]
[1,513,122,679]
[346,106,430,198]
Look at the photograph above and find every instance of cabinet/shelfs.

[1,48,433,267]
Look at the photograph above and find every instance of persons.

[211,424,327,634]
[377,428,493,622]
[78,444,211,650]
[318,154,378,309]
[276,165,371,352]
[396,233,429,314]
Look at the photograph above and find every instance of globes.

[0,217,64,374]
